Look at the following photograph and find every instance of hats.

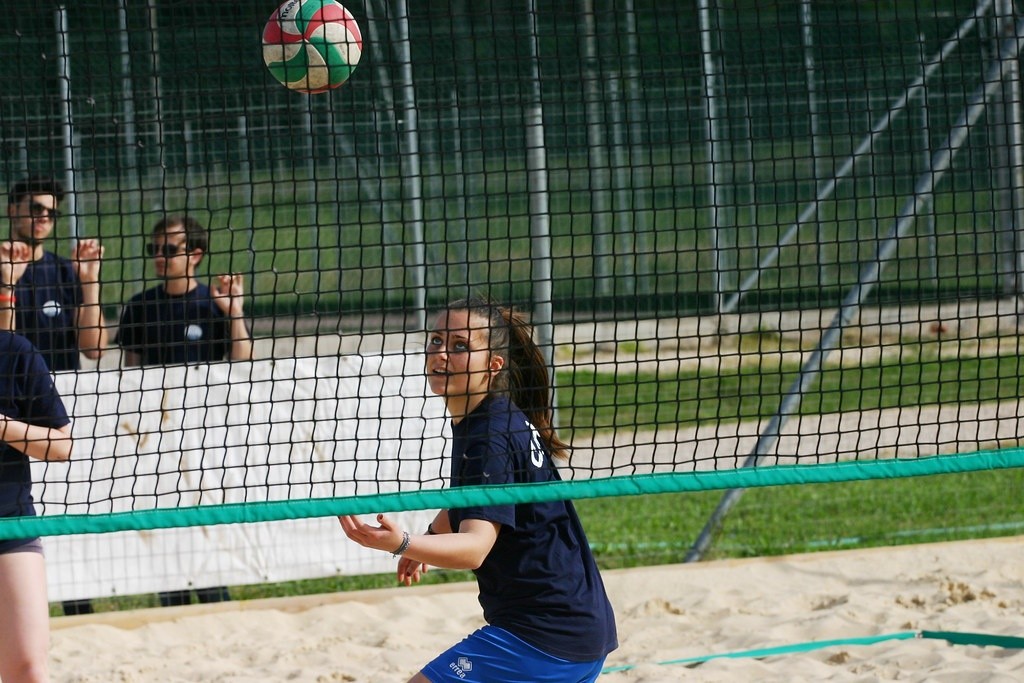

[7,176,64,204]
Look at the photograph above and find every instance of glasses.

[18,197,62,223]
[145,234,188,259]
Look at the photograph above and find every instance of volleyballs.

[262,0,363,95]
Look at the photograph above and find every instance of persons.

[0,173,108,615]
[0,330,76,683]
[339,298,619,683]
[115,216,252,606]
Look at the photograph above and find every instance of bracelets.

[428,523,435,535]
[0,295,16,303]
[391,531,410,558]
